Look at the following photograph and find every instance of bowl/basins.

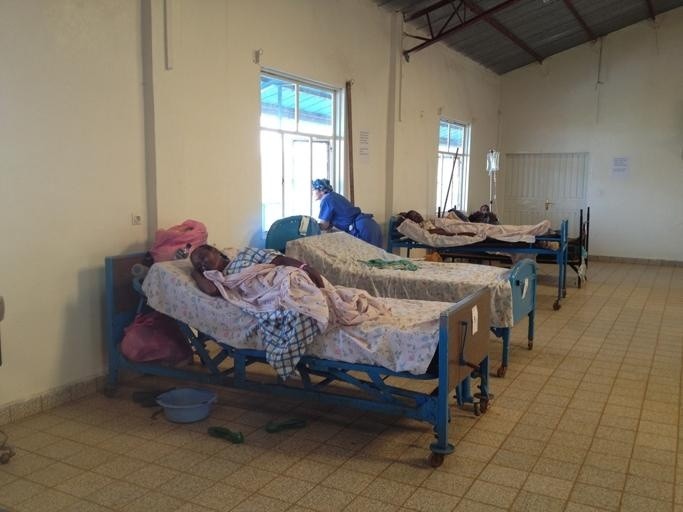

[154,388,218,423]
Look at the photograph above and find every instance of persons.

[189,244,325,297]
[405,210,478,238]
[312,178,383,248]
[468,204,500,226]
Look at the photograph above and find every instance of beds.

[107,250,493,467]
[265,216,538,379]
[387,212,568,311]
[450,207,589,288]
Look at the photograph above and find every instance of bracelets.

[298,264,308,270]
[456,233,459,236]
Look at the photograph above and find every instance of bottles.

[171,242,193,260]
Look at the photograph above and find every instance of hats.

[314,178,333,193]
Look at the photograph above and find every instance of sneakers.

[209,425,242,442]
[133,387,175,408]
[267,417,306,433]
